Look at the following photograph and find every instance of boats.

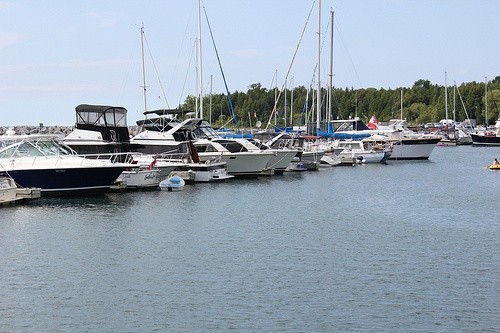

[0,0,500,202]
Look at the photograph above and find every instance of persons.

[492,157,500,164]
